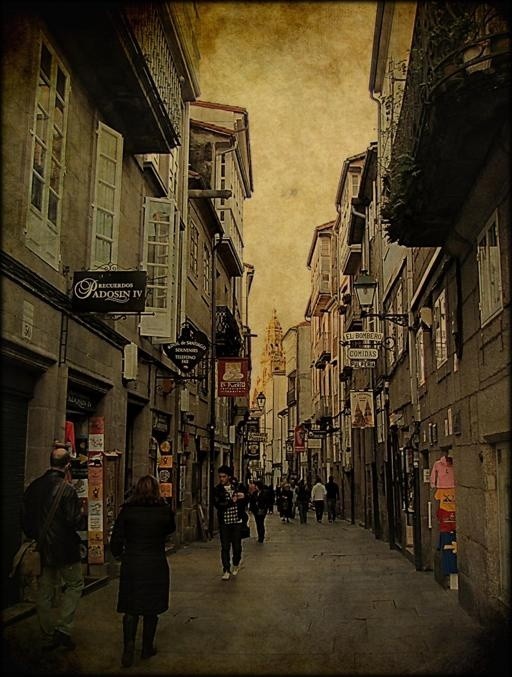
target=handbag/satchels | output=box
[9,539,41,578]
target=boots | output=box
[121,615,139,667]
[141,618,158,659]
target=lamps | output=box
[249,391,266,412]
[354,269,409,326]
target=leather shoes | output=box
[53,629,76,648]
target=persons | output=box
[211,473,343,545]
[211,462,250,582]
[108,472,178,666]
[21,445,88,648]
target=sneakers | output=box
[232,566,239,576]
[221,571,230,580]
